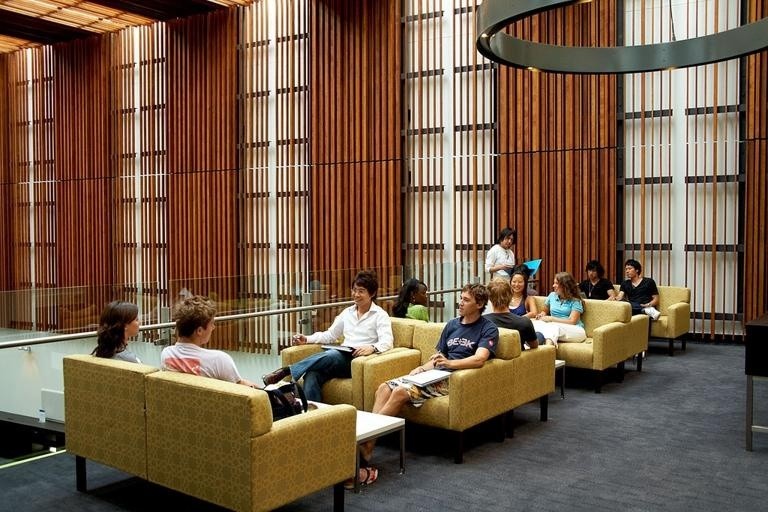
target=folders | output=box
[505,259,543,277]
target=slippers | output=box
[343,467,378,490]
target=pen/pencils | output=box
[293,335,298,344]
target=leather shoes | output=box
[262,368,286,386]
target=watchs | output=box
[648,303,652,307]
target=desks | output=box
[744,310,768,451]
[322,406,415,476]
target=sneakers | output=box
[644,307,660,320]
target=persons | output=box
[160,295,376,490]
[615,259,660,315]
[89,300,143,363]
[481,278,539,351]
[533,272,587,350]
[263,271,394,402]
[510,264,539,296]
[485,228,516,282]
[577,260,616,301]
[392,278,430,320]
[358,283,499,462]
[508,271,537,319]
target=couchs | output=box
[298,307,563,429]
[643,285,691,356]
[63,356,355,512]
[502,296,651,382]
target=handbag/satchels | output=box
[263,380,307,422]
